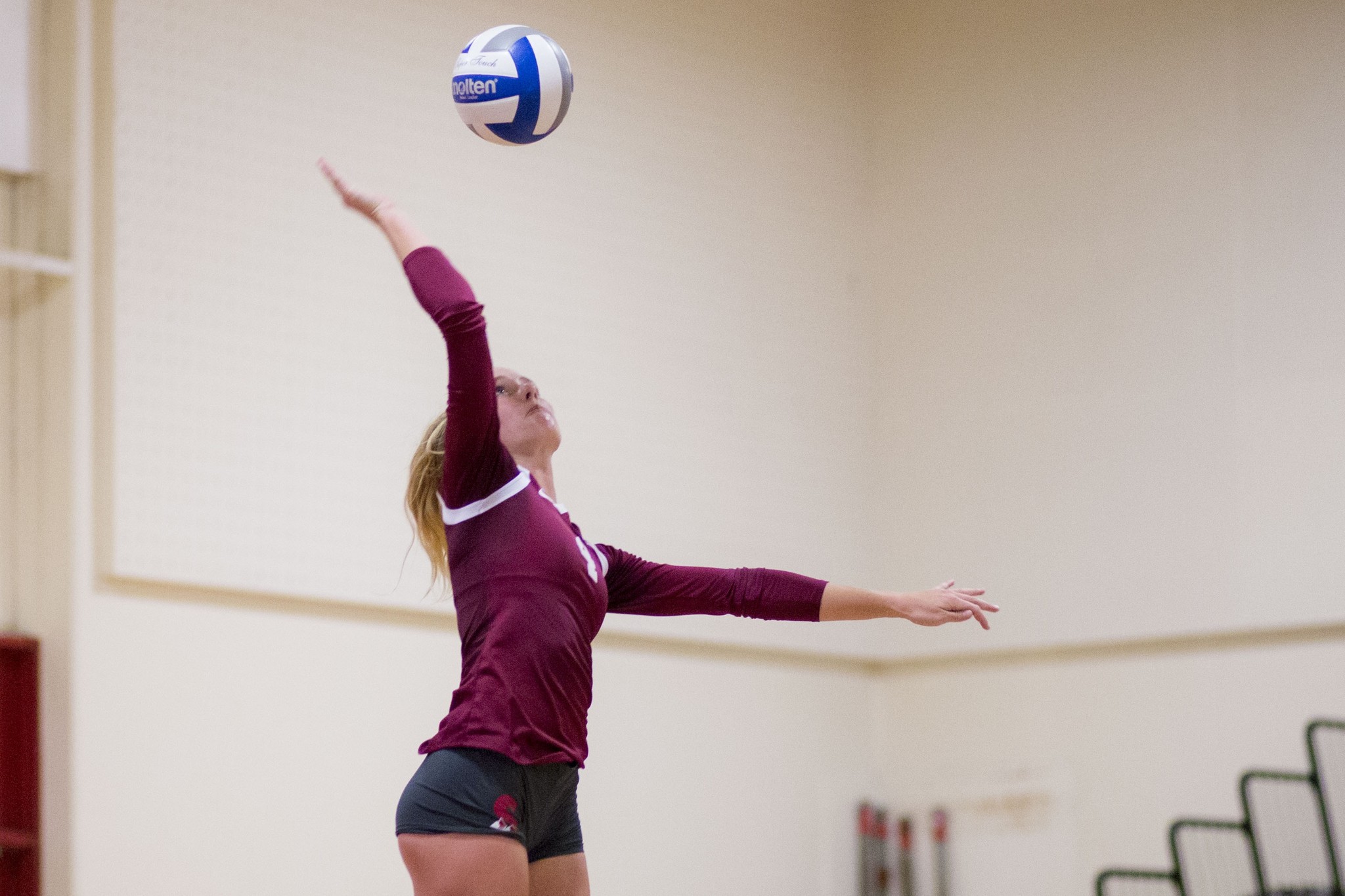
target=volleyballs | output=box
[451,24,573,146]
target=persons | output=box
[322,159,1000,896]
